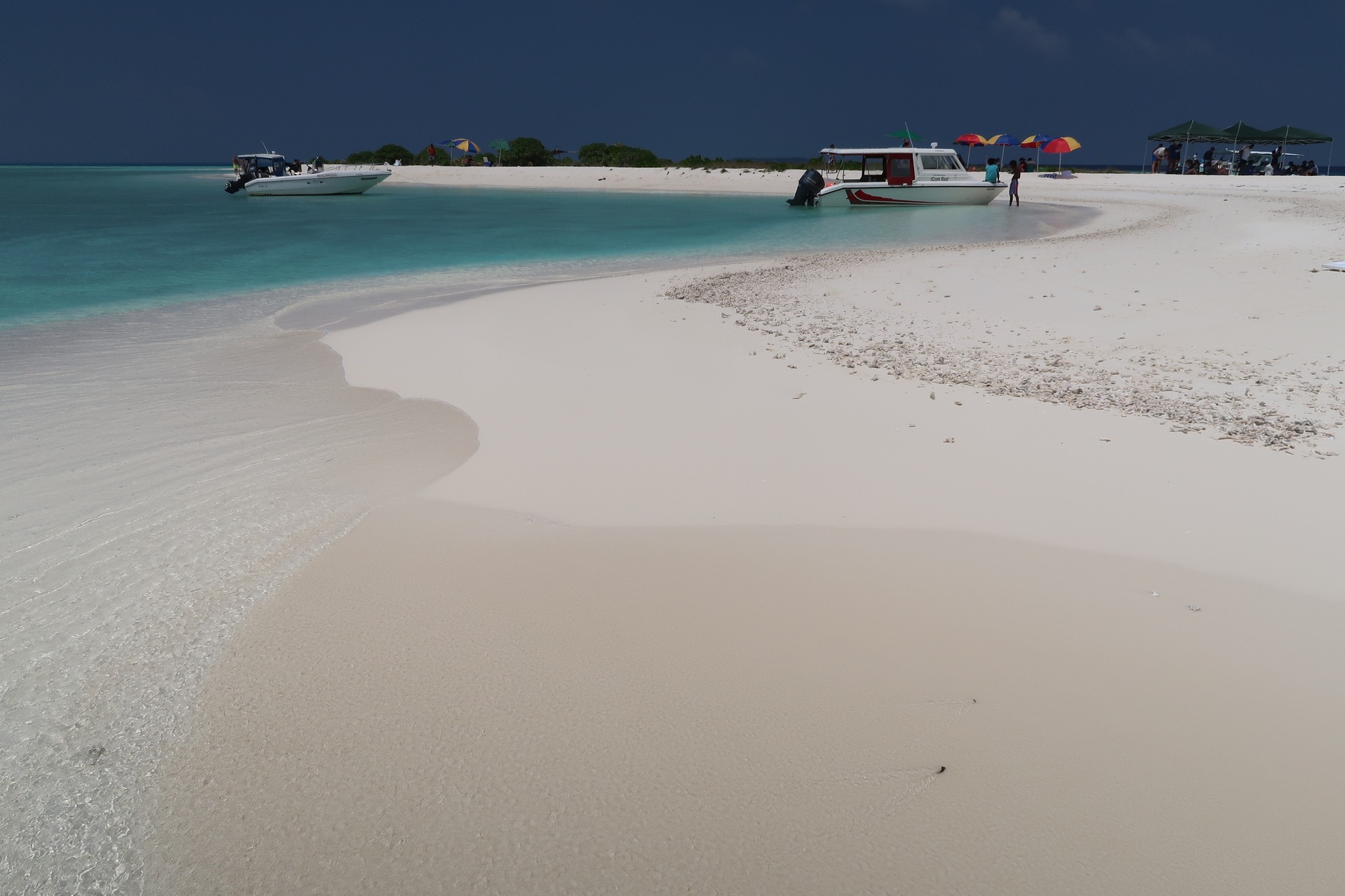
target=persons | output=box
[995,158,1004,171]
[1007,160,1021,206]
[233,156,238,175]
[826,144,838,174]
[957,154,965,168]
[427,144,438,167]
[1150,143,1318,175]
[293,159,304,176]
[985,158,1000,183]
[1017,157,1036,173]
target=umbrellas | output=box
[437,140,457,166]
[448,138,481,166]
[884,130,926,144]
[1020,134,1052,172]
[1039,137,1082,174]
[952,133,988,169]
[487,139,513,166]
[984,134,1021,172]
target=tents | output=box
[1142,120,1235,176]
[1266,126,1334,176]
[1221,121,1285,175]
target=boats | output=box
[1176,148,1304,175]
[223,139,392,196]
[784,121,1008,208]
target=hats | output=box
[1307,160,1314,163]
[1288,162,1293,166]
[1302,160,1307,164]
[1211,147,1216,150]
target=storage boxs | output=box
[483,157,491,167]
